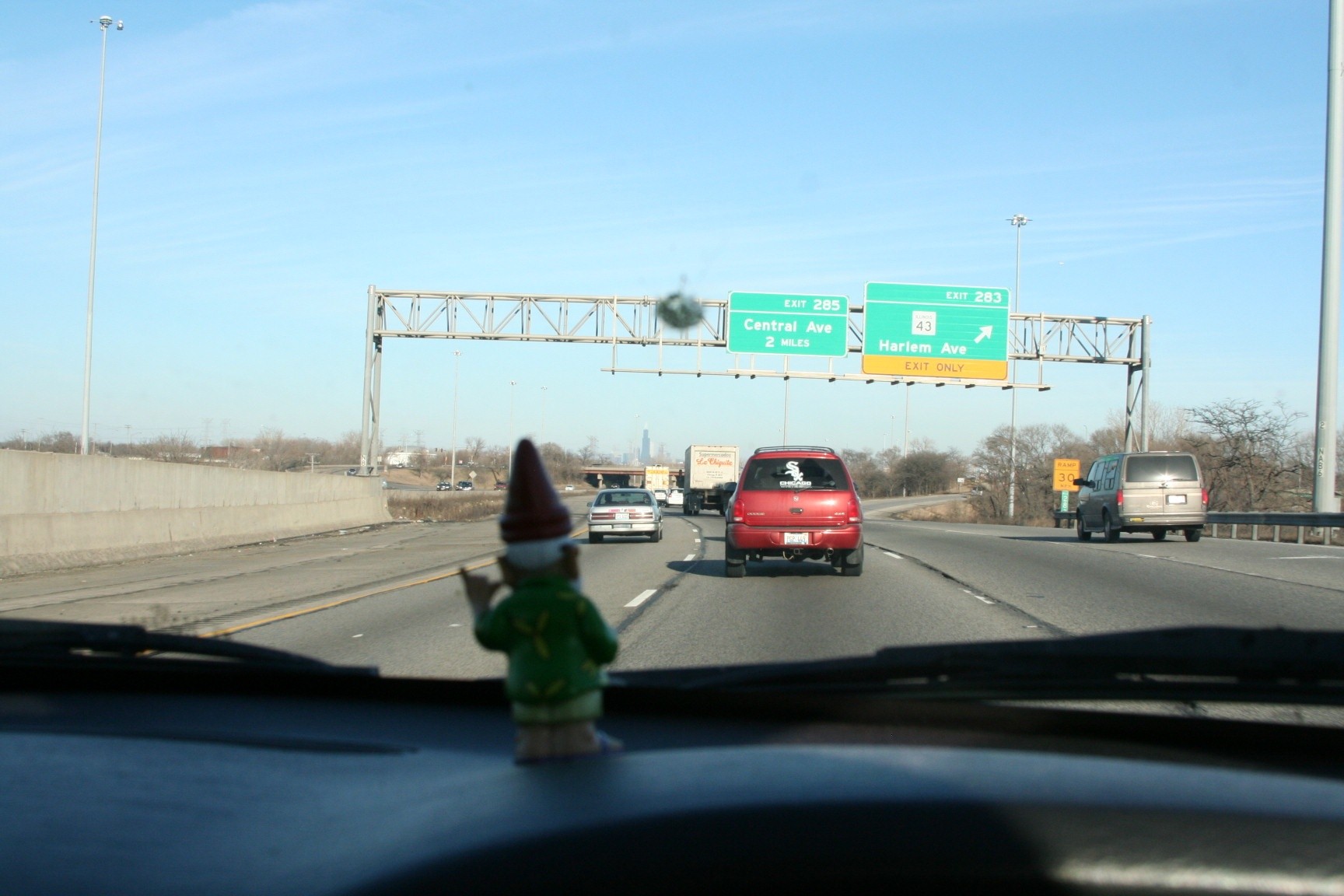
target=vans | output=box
[972,486,983,495]
[1073,450,1208,543]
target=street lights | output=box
[451,347,460,486]
[541,386,546,452]
[509,380,516,479]
[80,16,123,454]
[1006,216,1032,517]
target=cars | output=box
[397,464,403,468]
[718,481,738,515]
[587,487,664,543]
[565,485,574,490]
[349,468,355,474]
[494,481,509,490]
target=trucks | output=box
[679,444,739,515]
[644,463,669,490]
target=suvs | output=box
[666,488,685,507]
[653,489,667,505]
[724,444,864,577]
[436,481,474,491]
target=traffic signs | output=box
[862,282,1009,380]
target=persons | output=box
[461,439,623,763]
